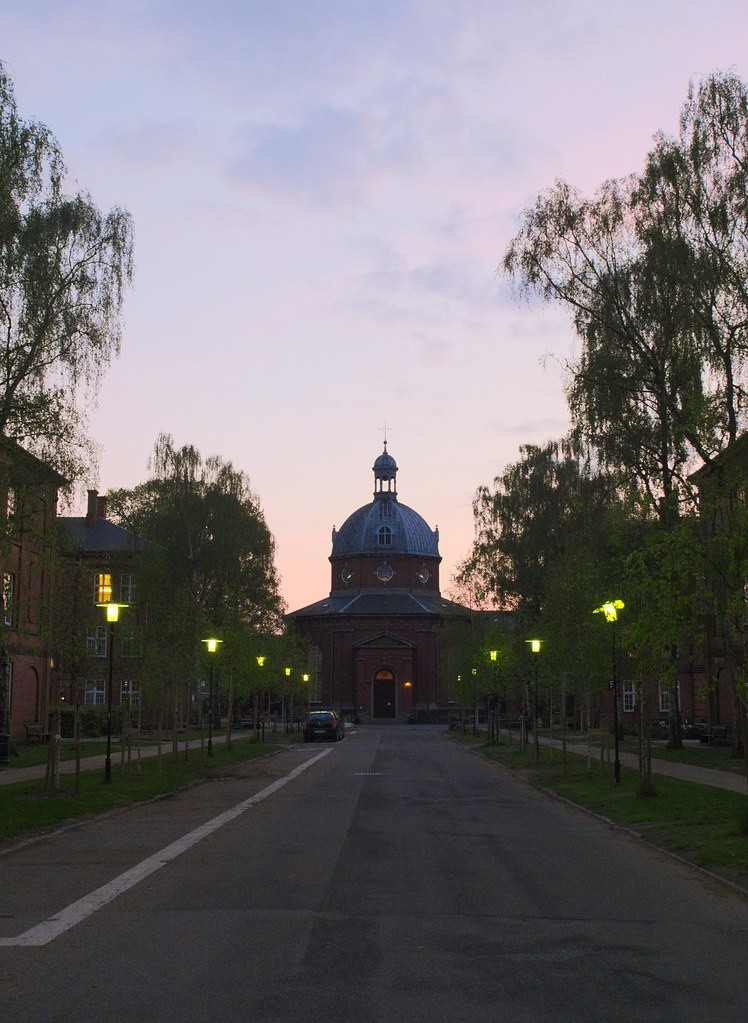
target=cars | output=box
[302,708,344,742]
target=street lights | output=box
[256,655,270,744]
[201,637,225,757]
[281,666,297,740]
[300,672,313,724]
[594,598,628,782]
[521,637,548,759]
[489,647,506,741]
[100,603,131,784]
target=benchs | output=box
[23,724,50,750]
[699,726,729,747]
